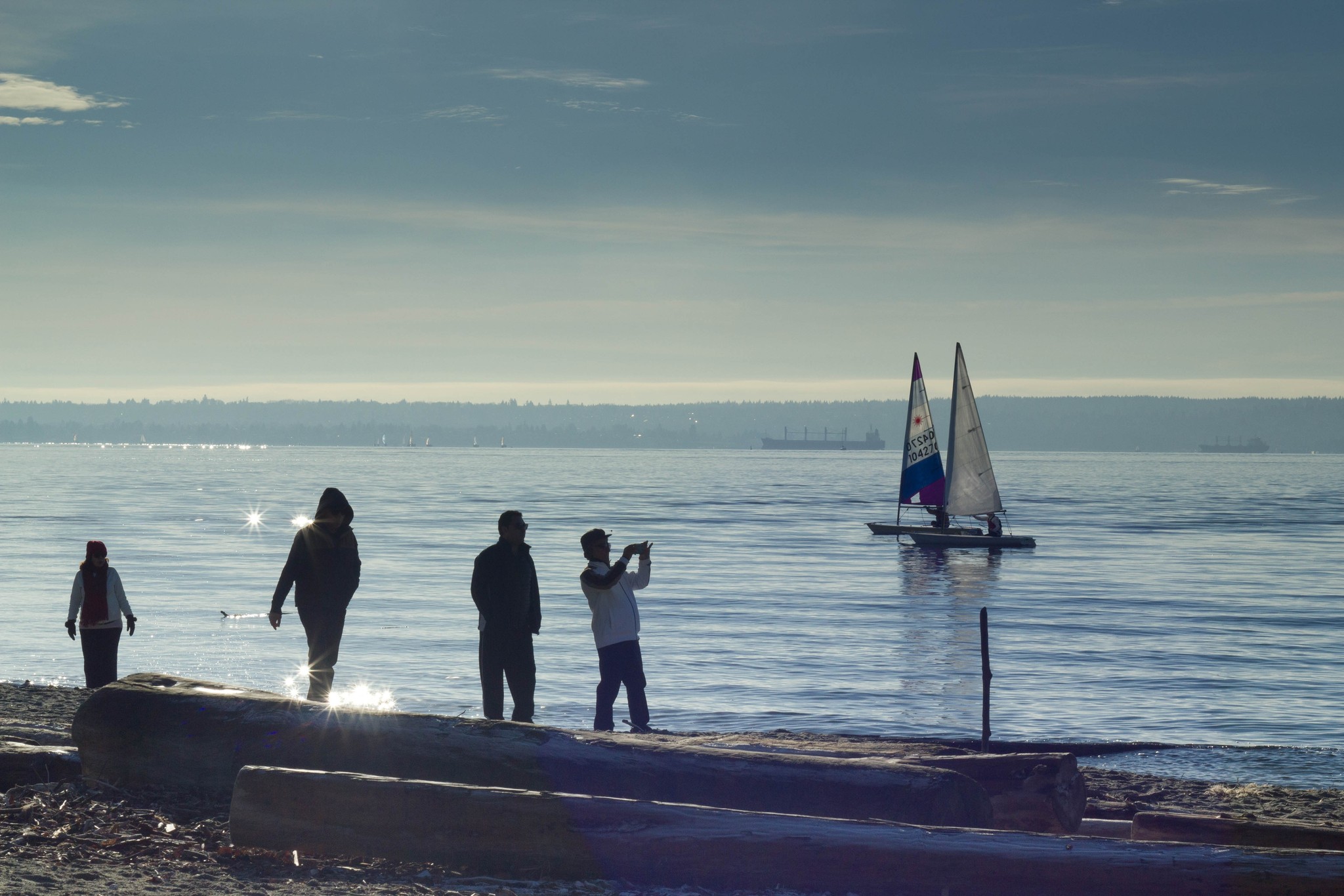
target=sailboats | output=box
[863,350,983,537]
[903,342,1038,550]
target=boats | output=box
[760,425,886,450]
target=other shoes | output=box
[631,726,652,732]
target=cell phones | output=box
[634,544,646,554]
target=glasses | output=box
[594,543,610,550]
[332,510,347,516]
[91,554,106,558]
[507,523,529,530]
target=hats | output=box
[580,529,612,548]
[86,541,107,557]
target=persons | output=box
[579,528,656,734]
[471,510,541,724]
[923,505,950,528]
[972,512,1003,537]
[65,541,137,689]
[268,487,361,702]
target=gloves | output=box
[126,615,137,636]
[65,620,76,641]
